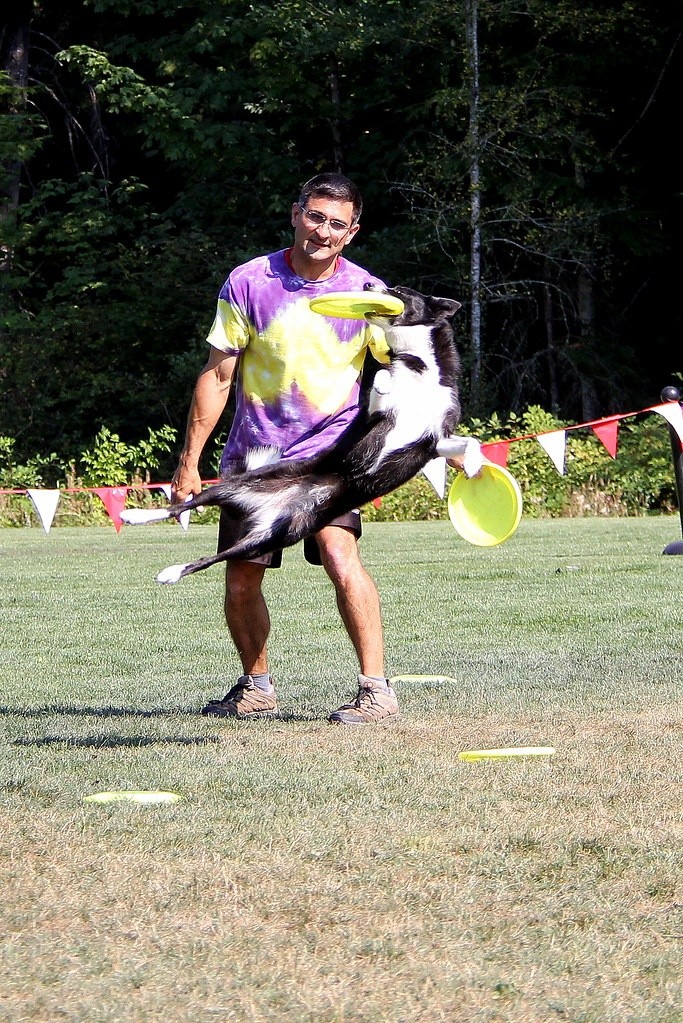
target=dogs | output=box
[118,282,482,588]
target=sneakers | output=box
[327,674,399,726]
[201,675,278,719]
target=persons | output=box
[171,173,398,723]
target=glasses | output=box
[299,207,351,231]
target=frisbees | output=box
[309,291,404,320]
[447,463,523,547]
